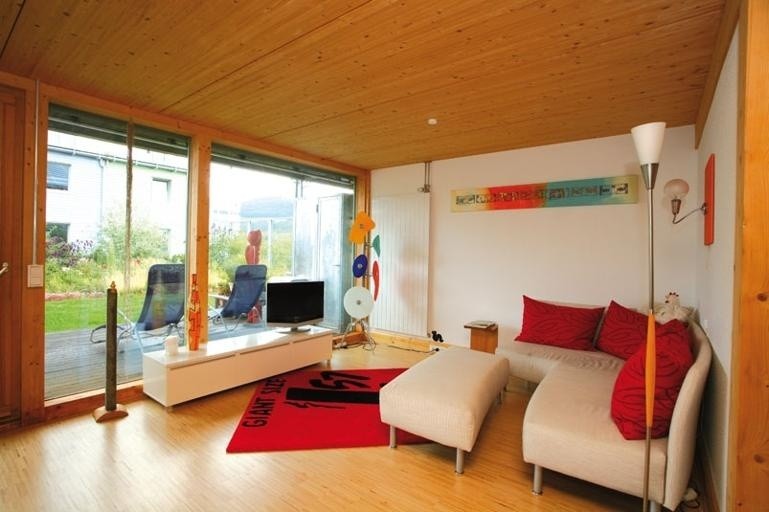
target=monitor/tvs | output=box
[266,281,324,334]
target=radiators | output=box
[364,192,431,339]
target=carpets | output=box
[227,367,435,453]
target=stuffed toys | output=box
[654,291,692,326]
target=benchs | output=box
[379,347,510,474]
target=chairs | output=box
[89,263,185,353]
[209,264,267,338]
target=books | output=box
[466,320,494,329]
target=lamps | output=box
[664,153,715,245]
[630,122,667,512]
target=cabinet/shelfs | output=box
[292,194,354,333]
[142,324,333,411]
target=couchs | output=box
[502,299,712,512]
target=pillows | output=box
[513,294,695,440]
